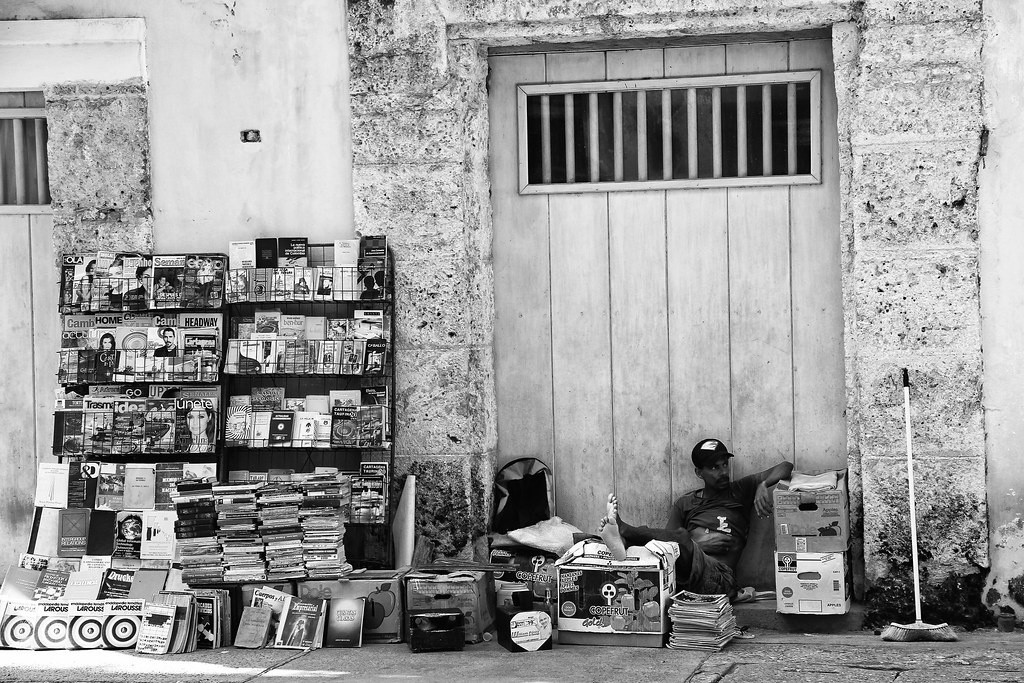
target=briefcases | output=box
[404,607,465,654]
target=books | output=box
[0,462,392,649]
[227,310,385,380]
[227,385,392,452]
[61,254,222,314]
[225,240,386,305]
[58,314,222,384]
[47,385,220,460]
[667,584,739,655]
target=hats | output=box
[691,438,735,467]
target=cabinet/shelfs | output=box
[57,243,397,585]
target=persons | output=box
[359,269,383,300]
[125,264,151,309]
[153,326,177,357]
[153,273,176,300]
[104,258,124,302]
[181,397,216,456]
[75,259,96,302]
[286,617,310,647]
[92,333,119,381]
[319,275,333,295]
[599,437,796,594]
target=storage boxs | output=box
[406,607,466,654]
[405,571,498,645]
[242,584,292,610]
[297,578,402,643]
[496,604,553,652]
[554,564,676,647]
[773,473,852,615]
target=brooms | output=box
[879,366,959,643]
